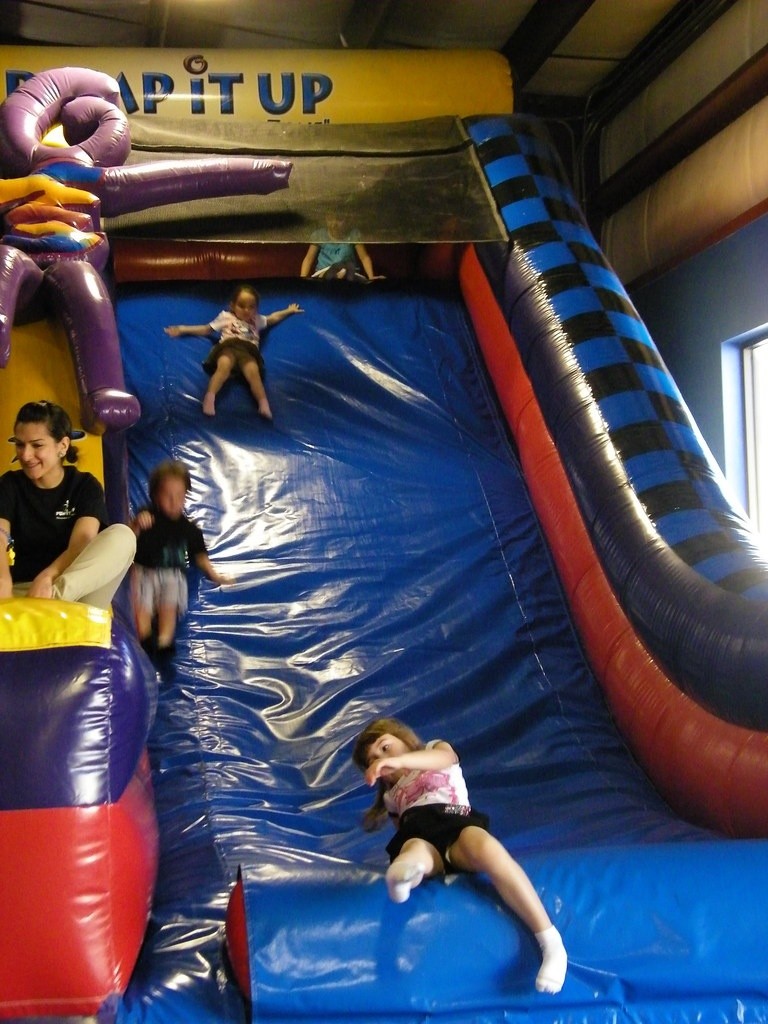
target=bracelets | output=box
[0,527,12,543]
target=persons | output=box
[164,284,304,419]
[299,204,387,284]
[0,400,136,609]
[131,460,233,678]
[352,717,568,994]
